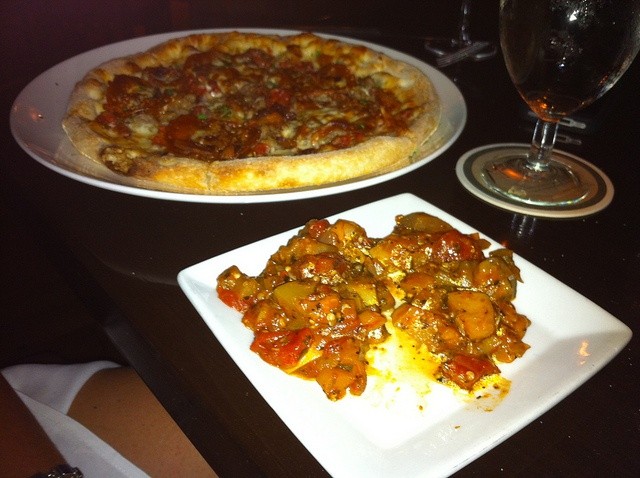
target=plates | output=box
[9,29,469,203]
[177,191,633,478]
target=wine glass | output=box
[480,0,639,208]
[424,0,497,62]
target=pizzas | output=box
[60,30,441,194]
